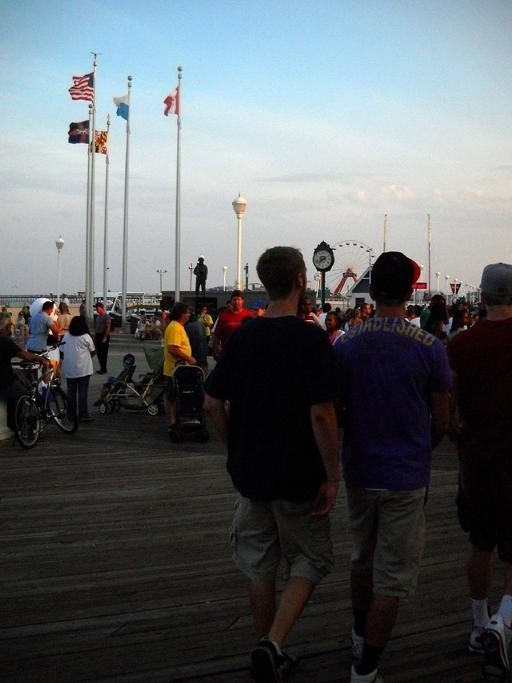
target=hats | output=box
[93,301,105,308]
[479,261,512,294]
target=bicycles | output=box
[15,340,78,448]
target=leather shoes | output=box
[97,370,100,372]
[100,367,106,374]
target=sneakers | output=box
[252,635,290,682]
[479,612,509,683]
[351,637,364,661]
[467,623,486,654]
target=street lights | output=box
[454,278,475,303]
[435,270,440,292]
[231,191,248,291]
[222,264,228,290]
[157,267,167,291]
[419,264,423,301]
[445,274,448,304]
[188,262,195,291]
[54,234,66,306]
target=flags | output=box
[68,120,89,143]
[163,86,180,116]
[90,130,108,154]
[114,98,129,120]
[69,73,94,102]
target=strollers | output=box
[93,337,165,416]
[168,357,209,442]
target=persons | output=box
[446,262,512,681]
[94,294,488,446]
[203,246,342,683]
[331,252,452,682]
[1,298,110,440]
[193,258,208,295]
